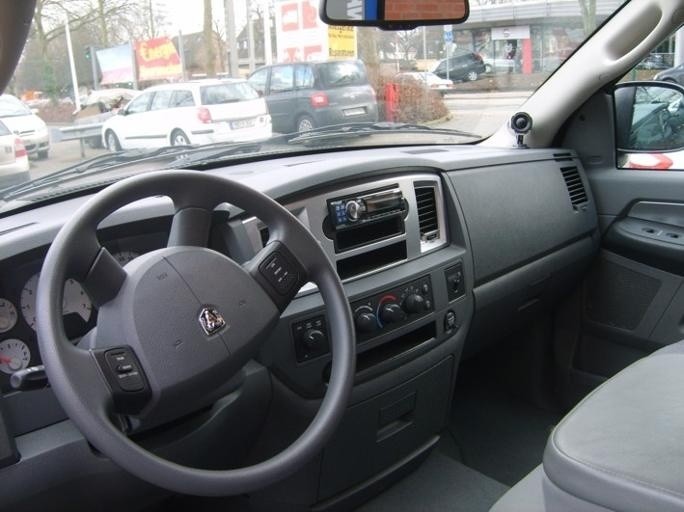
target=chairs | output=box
[544,337,683,512]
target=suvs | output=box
[433,53,486,81]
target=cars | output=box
[0,94,51,187]
[390,69,453,98]
[652,62,684,87]
[101,76,273,159]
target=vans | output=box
[247,58,378,146]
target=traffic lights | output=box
[84,46,91,59]
